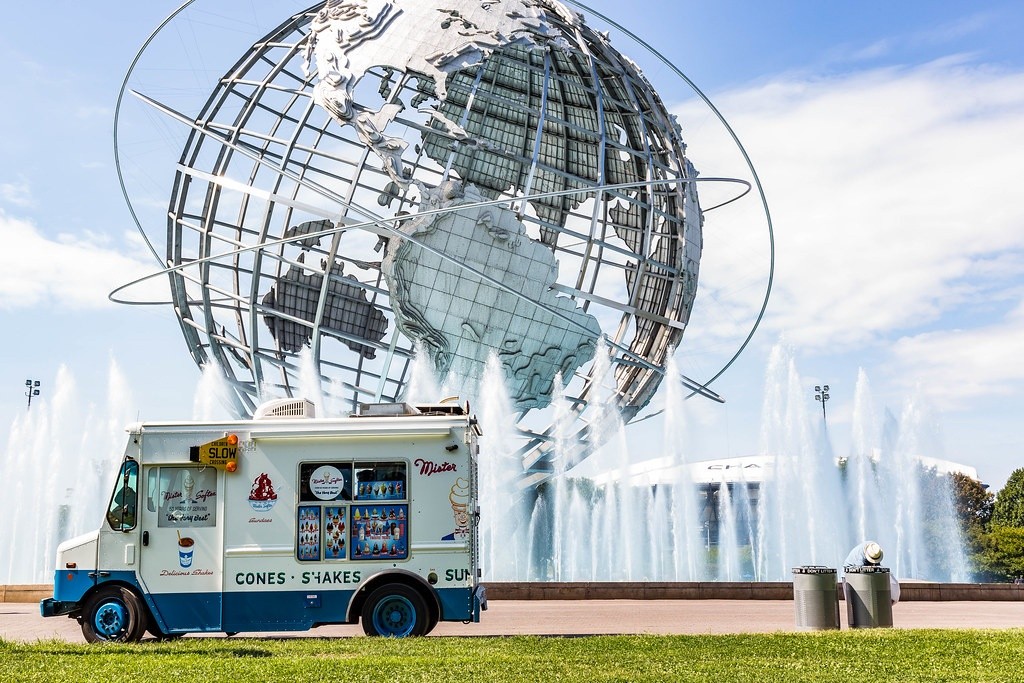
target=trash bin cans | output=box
[844,564,894,628]
[792,563,840,633]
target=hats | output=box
[864,543,883,563]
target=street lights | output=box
[815,383,830,423]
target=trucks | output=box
[38,394,489,647]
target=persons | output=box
[842,541,883,593]
